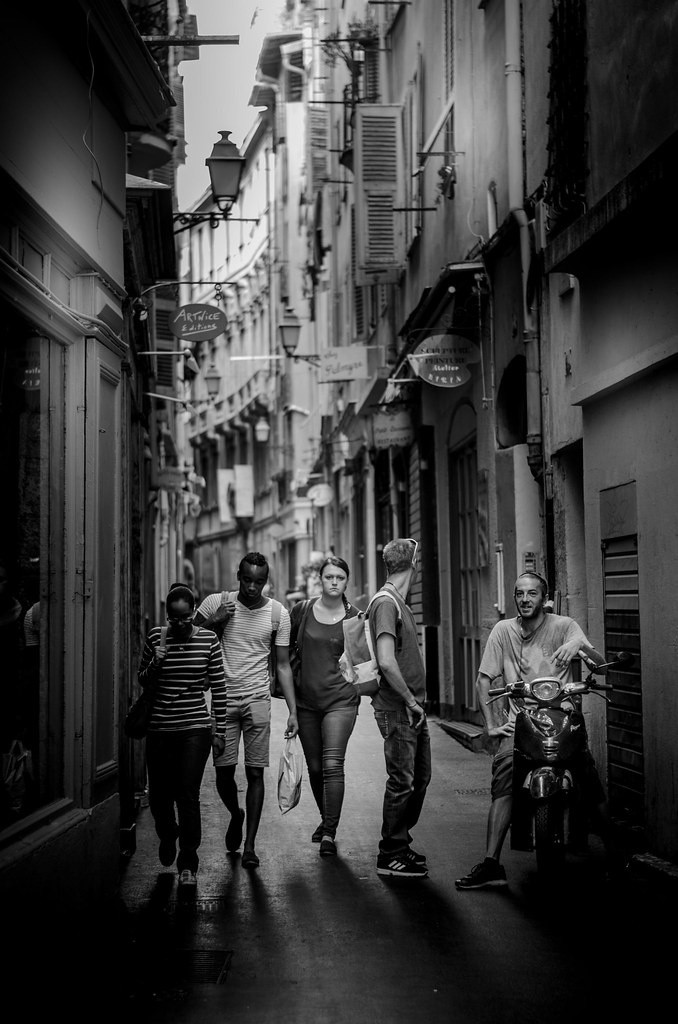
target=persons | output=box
[192,552,298,867]
[287,556,366,857]
[362,537,432,876]
[137,583,226,887]
[0,565,41,648]
[454,572,608,889]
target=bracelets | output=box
[214,734,226,739]
[409,703,417,708]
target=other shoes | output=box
[242,851,259,866]
[226,808,245,851]
[321,840,336,855]
[311,825,323,842]
[159,822,178,867]
[177,847,199,875]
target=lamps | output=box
[172,130,247,235]
[277,306,322,368]
[255,415,294,458]
[174,362,223,415]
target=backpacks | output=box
[339,590,401,695]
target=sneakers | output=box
[377,855,428,877]
[455,857,507,890]
[405,849,426,865]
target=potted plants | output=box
[347,16,381,38]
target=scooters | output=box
[482,648,636,898]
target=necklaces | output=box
[386,582,405,601]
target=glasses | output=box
[406,539,418,563]
[167,615,192,623]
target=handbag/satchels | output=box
[271,600,312,699]
[277,732,304,814]
[124,688,154,739]
